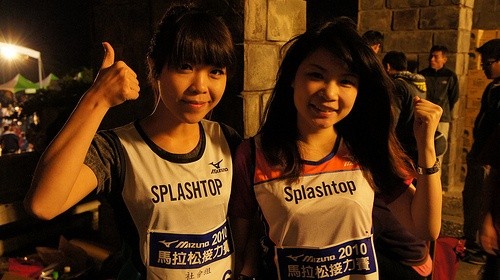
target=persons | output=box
[417,45,459,180]
[455,38,500,265]
[1,89,39,151]
[382,50,426,188]
[362,30,384,54]
[477,164,500,280]
[228,15,443,280]
[22,2,260,280]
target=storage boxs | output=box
[459,29,500,180]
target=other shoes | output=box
[468,253,488,265]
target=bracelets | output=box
[418,158,441,176]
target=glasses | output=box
[480,60,498,67]
